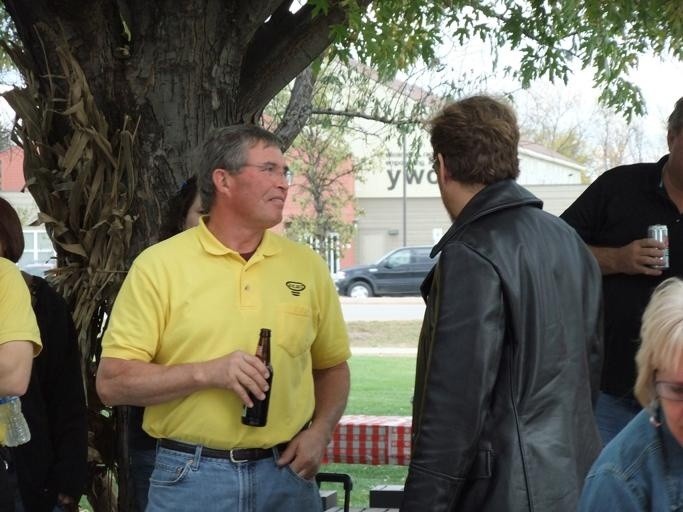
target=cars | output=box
[334,245,441,298]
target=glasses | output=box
[241,165,294,179]
[655,382,683,400]
[432,158,440,174]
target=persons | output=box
[399,95,603,512]
[577,277,683,512]
[0,256,43,512]
[558,97,683,449]
[95,124,351,512]
[0,197,89,512]
[132,175,203,512]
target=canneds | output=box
[647,224,670,270]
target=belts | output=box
[159,438,290,463]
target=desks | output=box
[327,414,415,466]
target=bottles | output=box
[0,392,32,448]
[239,328,274,426]
